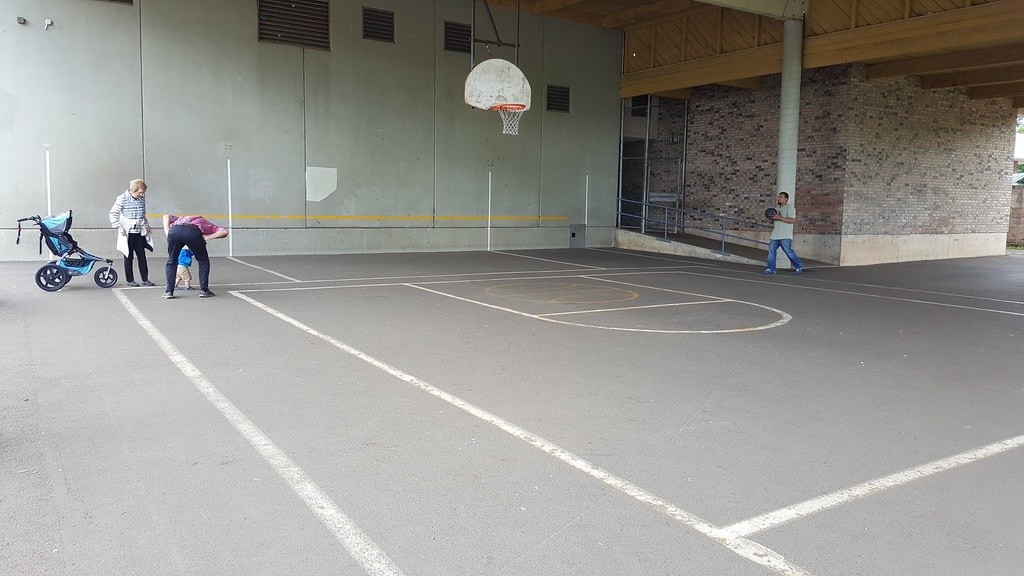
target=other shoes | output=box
[185,286,194,290]
[174,286,180,289]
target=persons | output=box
[108,179,156,286]
[162,214,228,299]
[762,192,805,274]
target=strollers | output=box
[17,207,117,292]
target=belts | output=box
[174,223,194,225]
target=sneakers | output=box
[763,269,776,274]
[199,289,215,296]
[162,292,173,299]
[142,280,154,286]
[128,281,139,287]
[795,266,804,275]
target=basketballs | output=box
[765,208,777,219]
